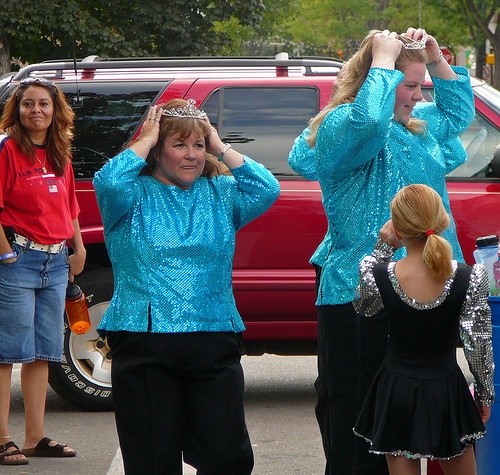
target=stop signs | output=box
[440,47,452,65]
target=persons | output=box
[352,184,495,475]
[0,77,86,465]
[92,98,280,475]
[288,27,476,475]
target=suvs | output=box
[1,51,499,411]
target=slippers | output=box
[21,437,76,457]
[0,441,29,465]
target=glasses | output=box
[20,77,54,86]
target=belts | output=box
[9,233,66,254]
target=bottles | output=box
[473,234,500,297]
[66,279,91,335]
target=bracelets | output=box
[218,143,231,161]
[0,252,17,260]
[426,50,443,67]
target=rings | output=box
[148,117,155,120]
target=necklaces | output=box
[35,153,47,174]
